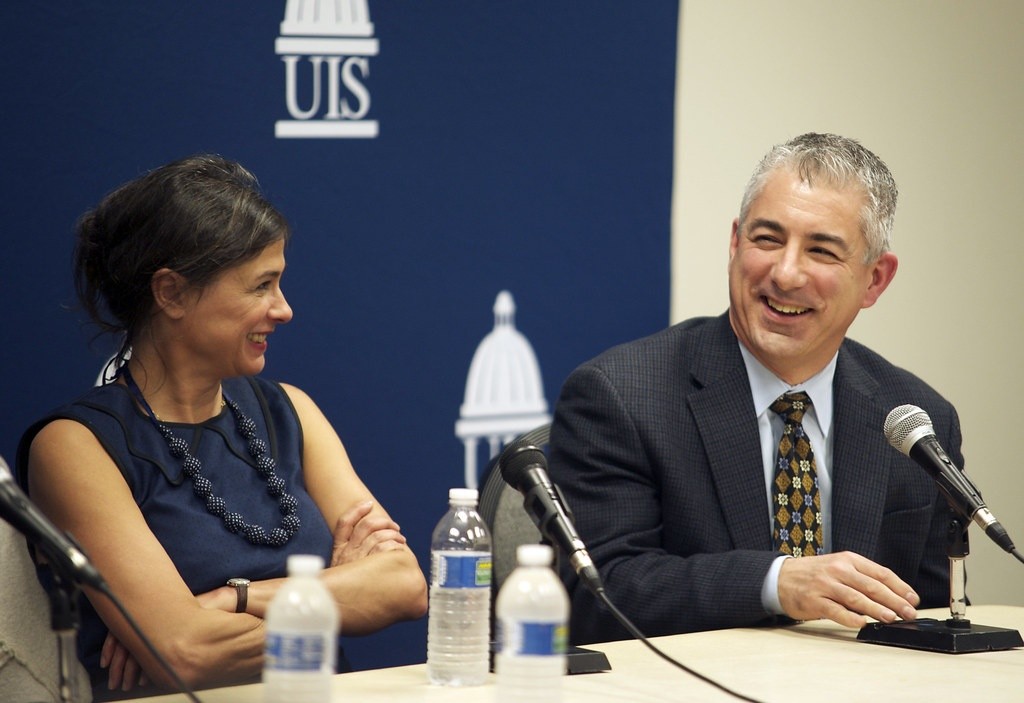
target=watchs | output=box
[227,577,251,613]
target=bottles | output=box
[497,544,568,703]
[263,555,338,703]
[427,488,492,688]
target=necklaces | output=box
[121,360,301,545]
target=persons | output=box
[546,132,971,644]
[10,154,429,703]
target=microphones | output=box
[497,438,606,598]
[0,454,103,591]
[883,404,1016,554]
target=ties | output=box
[768,391,826,557]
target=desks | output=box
[118,604,1024,703]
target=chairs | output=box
[0,417,554,703]
